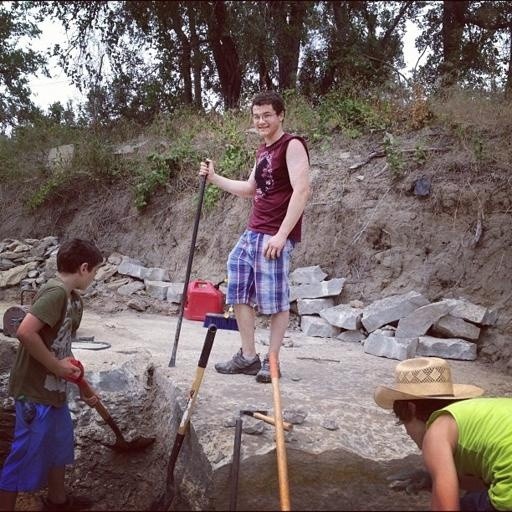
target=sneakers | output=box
[215,349,261,375]
[256,359,281,382]
[45,495,93,512]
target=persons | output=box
[372,357,512,512]
[0,237,104,512]
[199,93,311,383]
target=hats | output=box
[375,357,484,410]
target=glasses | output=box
[251,113,276,121]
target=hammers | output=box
[240,410,293,432]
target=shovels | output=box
[62,359,157,450]
[155,323,217,512]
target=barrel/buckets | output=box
[182,279,226,321]
